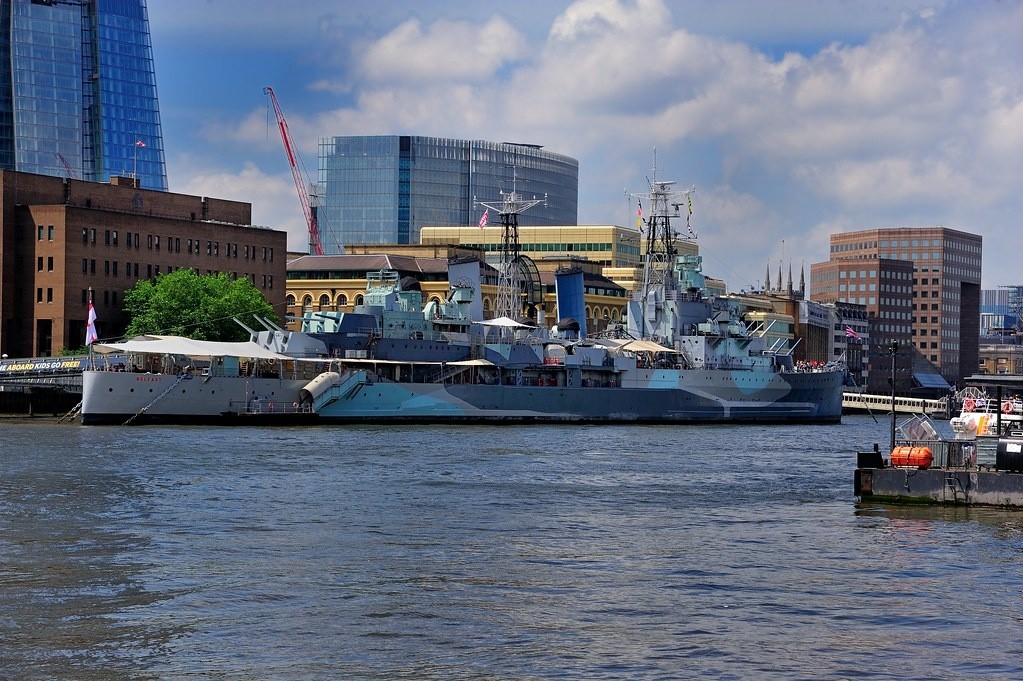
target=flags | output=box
[136,136,146,148]
[479,209,489,227]
[846,325,861,341]
[949,385,956,392]
[637,199,646,232]
[688,199,692,213]
[85,302,98,346]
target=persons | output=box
[269,401,274,412]
[797,360,825,373]
[292,401,300,410]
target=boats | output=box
[891,445,935,470]
[78,146,848,429]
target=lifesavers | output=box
[965,400,975,411]
[269,402,275,409]
[293,402,299,408]
[433,313,439,320]
[1003,402,1014,413]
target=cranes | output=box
[263,86,341,255]
